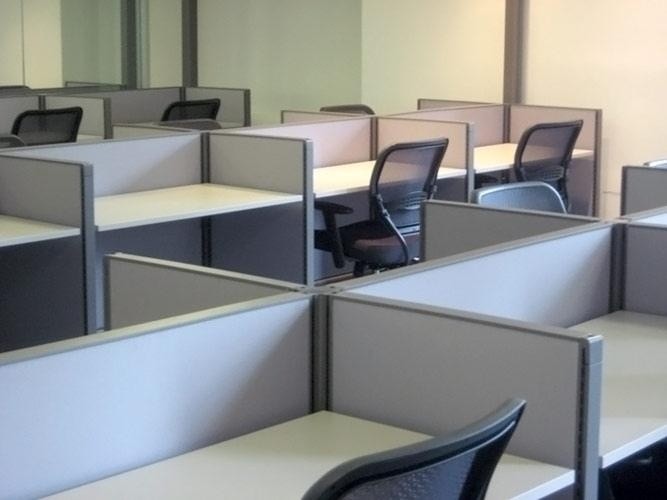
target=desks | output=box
[0,76,666,500]
